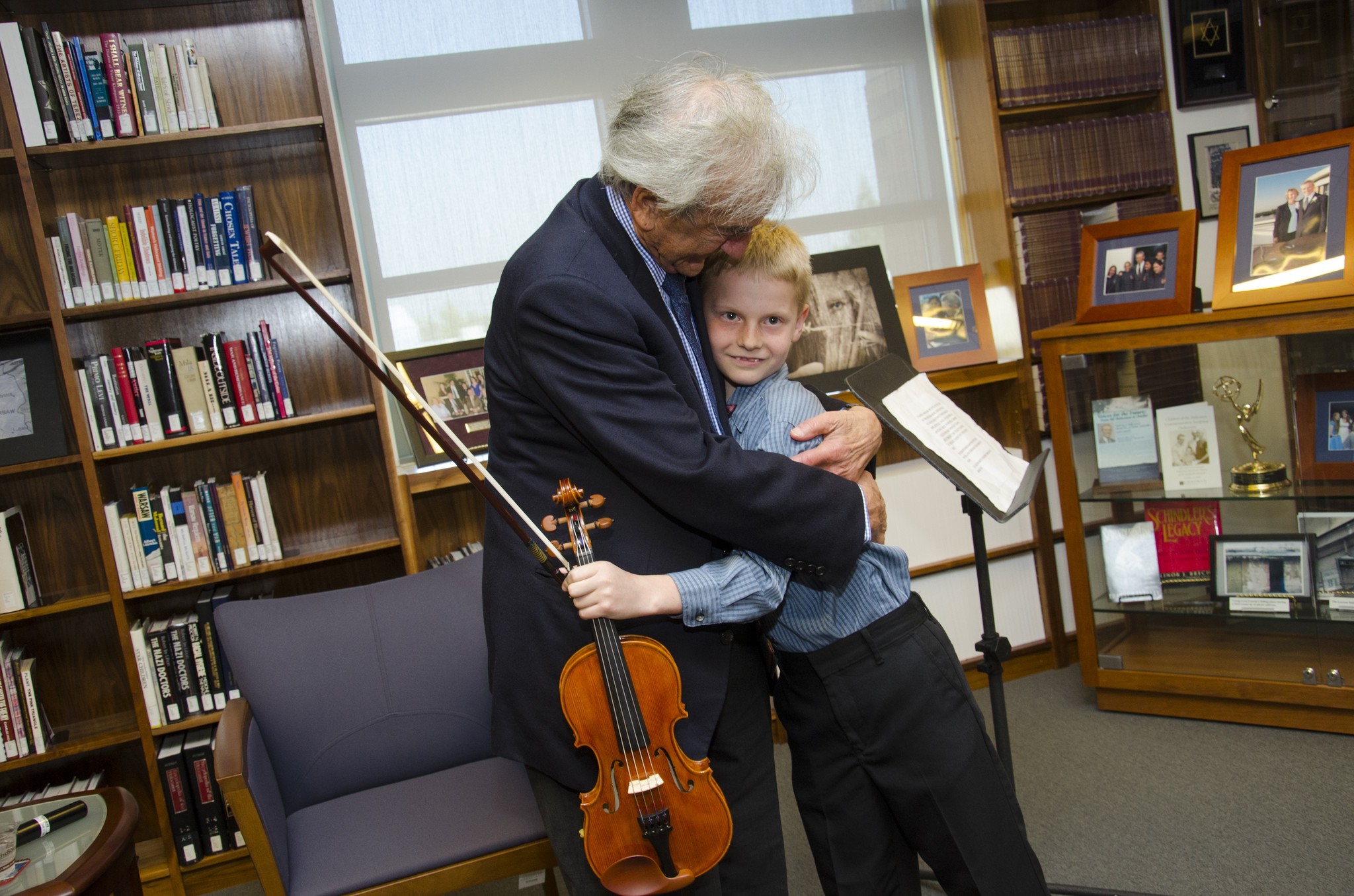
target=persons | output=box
[1169,430,1213,467]
[1329,410,1353,450]
[435,373,492,418]
[788,269,890,378]
[479,55,889,894]
[1106,249,1165,293]
[1295,180,1328,238]
[921,292,966,340]
[558,219,1051,896]
[1099,423,1116,442]
[1272,188,1300,244]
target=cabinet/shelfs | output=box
[934,0,1197,331]
[0,1,421,896]
[1029,295,1354,741]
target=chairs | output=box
[212,550,551,896]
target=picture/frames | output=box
[1208,532,1319,603]
[1210,128,1354,311]
[1065,202,1201,328]
[1167,0,1253,112]
[1187,125,1252,222]
[1290,362,1354,499]
[377,338,511,472]
[1265,0,1354,101]
[1273,113,1338,142]
[890,264,1000,373]
[781,246,911,392]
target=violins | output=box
[541,478,734,896]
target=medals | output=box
[1212,375,1293,493]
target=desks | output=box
[392,355,1073,749]
[0,783,140,896]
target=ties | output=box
[662,272,726,438]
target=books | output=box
[71,319,295,452]
[0,17,219,149]
[156,723,250,868]
[128,594,275,731]
[0,639,53,764]
[0,468,289,613]
[47,182,269,310]
